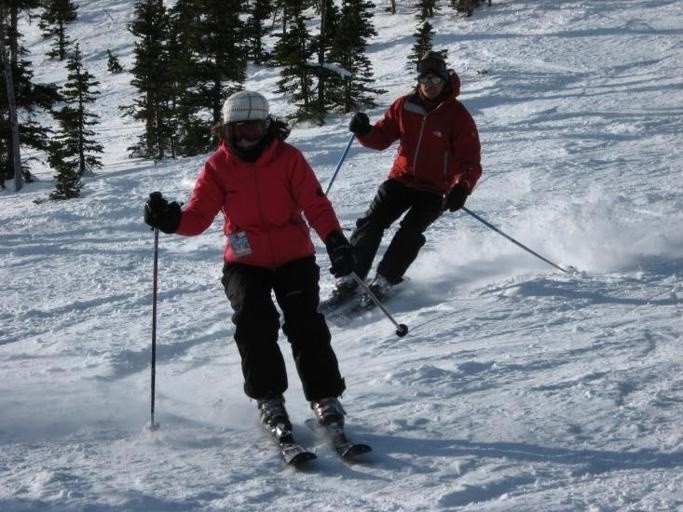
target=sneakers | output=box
[314,401,343,424]
[261,402,291,438]
[358,276,389,309]
[335,276,358,292]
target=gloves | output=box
[326,232,354,278]
[442,182,467,212]
[145,192,180,234]
[350,112,368,133]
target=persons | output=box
[144,91,359,438]
[336,51,483,308]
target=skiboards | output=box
[255,398,373,465]
[317,274,406,320]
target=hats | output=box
[419,52,446,78]
[224,91,270,122]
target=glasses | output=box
[222,123,265,141]
[418,77,442,83]
